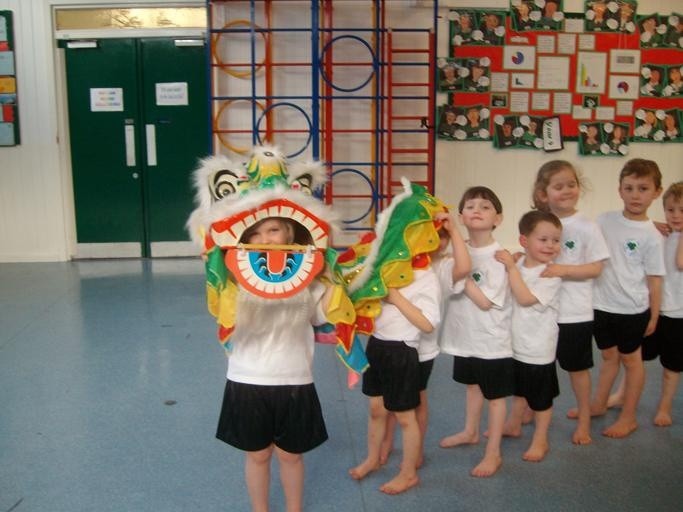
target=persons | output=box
[348,259,440,494]
[377,216,470,476]
[441,0,682,153]
[212,218,335,512]
[511,162,608,446]
[565,158,662,441]
[480,215,563,462]
[607,182,681,426]
[437,184,509,479]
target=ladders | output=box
[388,28,435,208]
